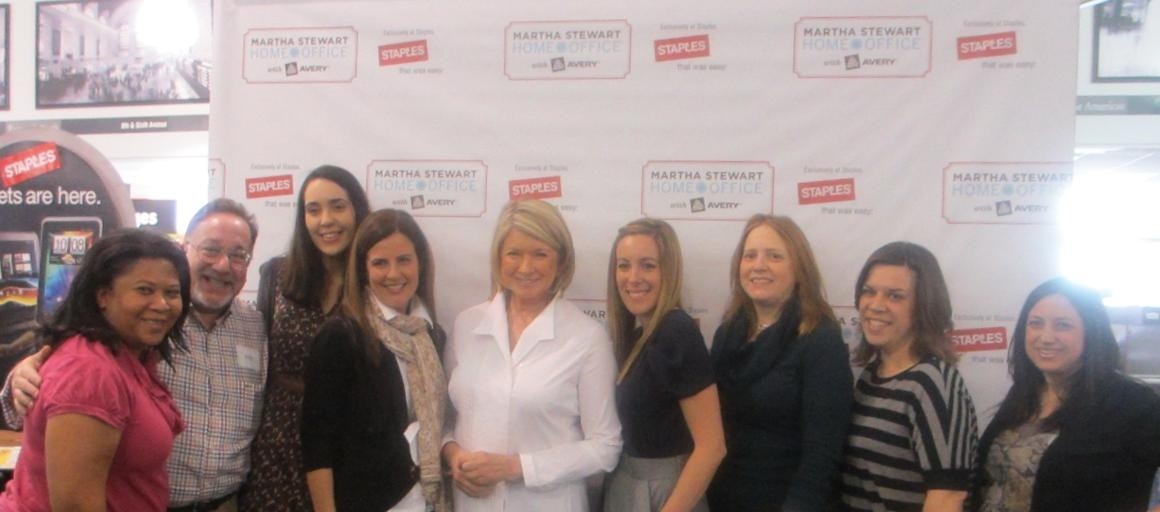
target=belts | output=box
[166,492,235,512]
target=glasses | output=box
[197,240,251,264]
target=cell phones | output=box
[35,215,103,322]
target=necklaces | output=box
[755,317,771,339]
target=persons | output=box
[979,275,1160,512]
[440,196,625,511]
[709,212,854,511]
[0,197,270,511]
[238,165,371,511]
[589,217,728,512]
[839,240,980,511]
[299,207,448,511]
[0,227,191,510]
